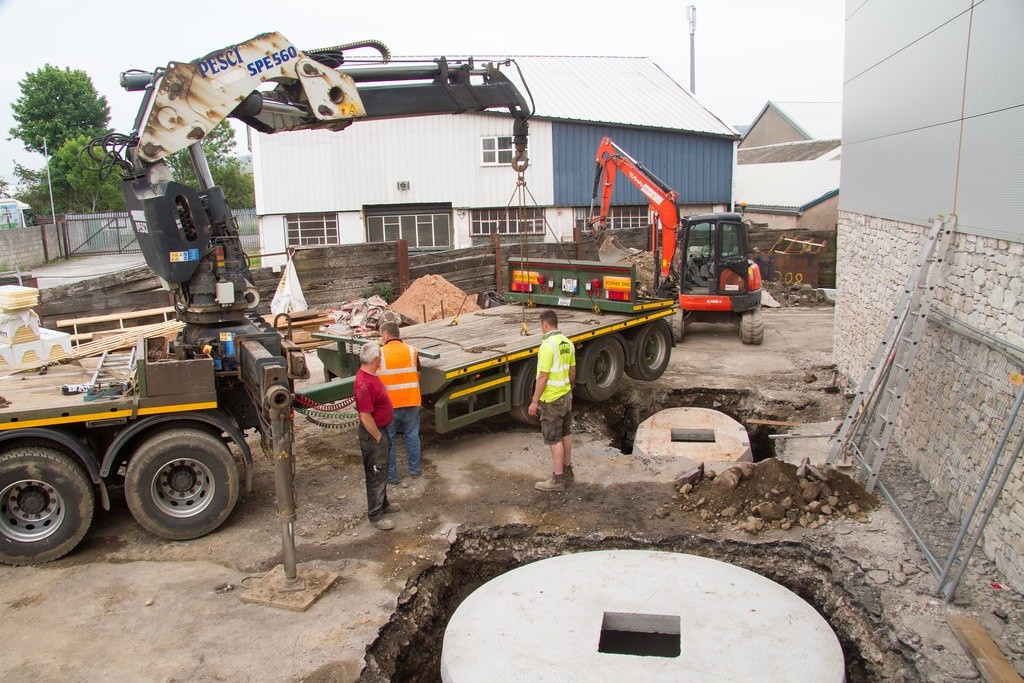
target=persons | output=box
[527,310,576,492]
[355,343,400,530]
[378,322,423,486]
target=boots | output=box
[563,463,574,482]
[535,472,565,491]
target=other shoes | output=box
[412,473,421,479]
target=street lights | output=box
[686,4,698,96]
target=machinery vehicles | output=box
[583,135,762,348]
[0,29,535,614]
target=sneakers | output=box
[370,517,395,529]
[382,503,401,513]
